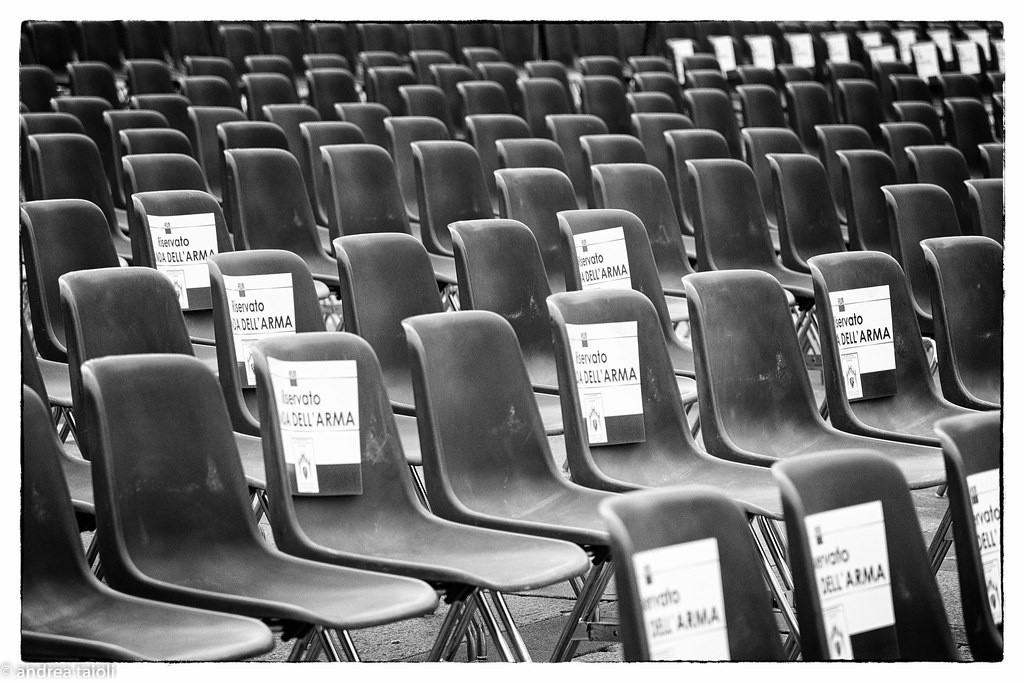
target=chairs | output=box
[19,20,1004,664]
